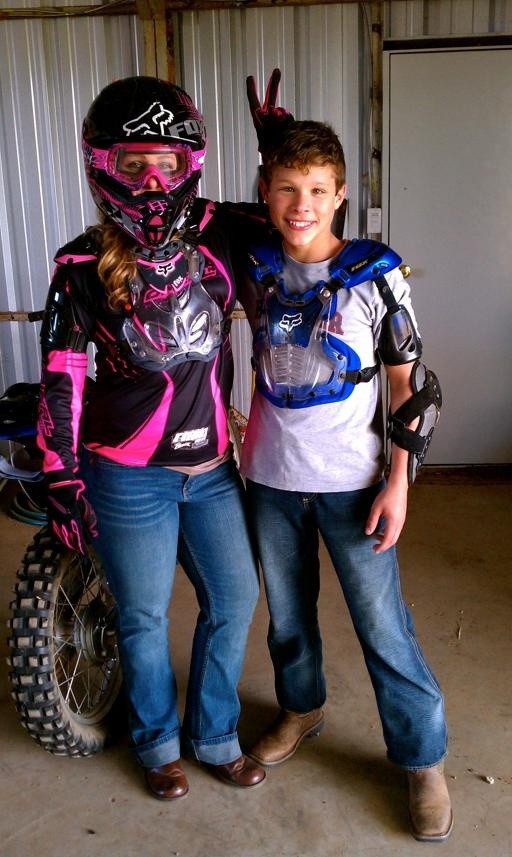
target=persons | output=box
[32,66,295,807]
[238,117,458,843]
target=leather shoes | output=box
[206,754,267,790]
[245,706,325,764]
[145,759,191,803]
[406,759,455,845]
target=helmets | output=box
[81,74,206,262]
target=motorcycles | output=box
[0,304,257,761]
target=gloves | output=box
[245,67,294,166]
[46,478,99,557]
